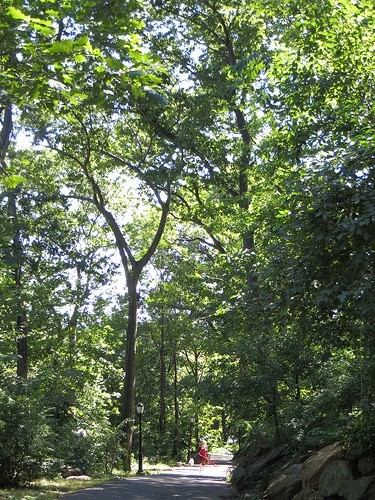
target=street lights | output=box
[136,402,146,477]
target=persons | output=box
[198,441,208,472]
